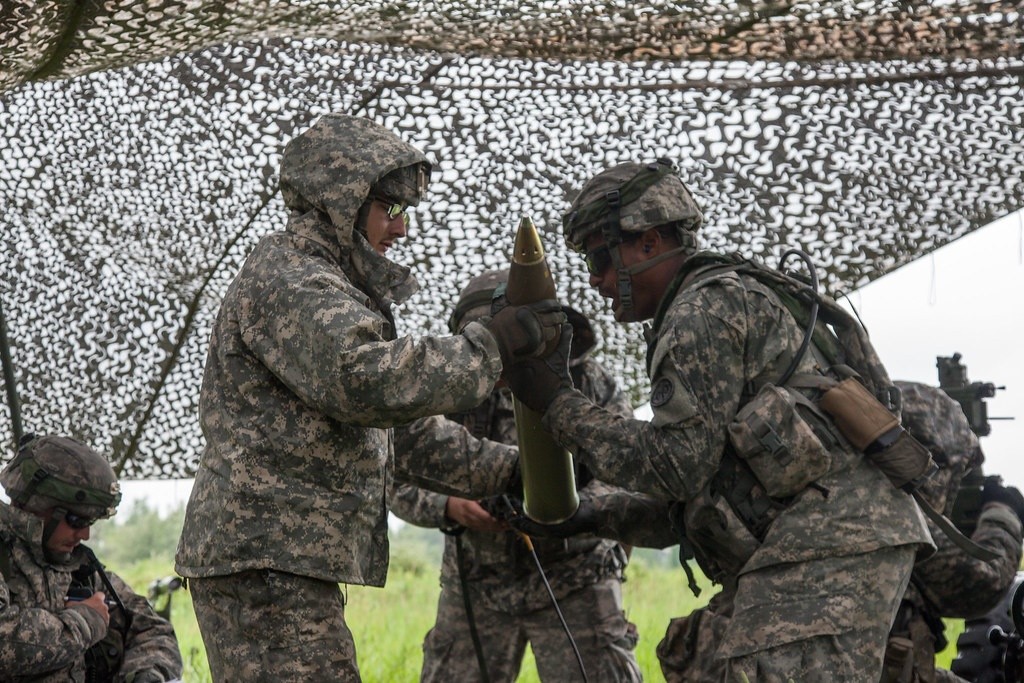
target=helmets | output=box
[449,266,511,336]
[562,157,703,254]
[367,159,430,207]
[0,433,122,518]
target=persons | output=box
[176,113,568,683]
[385,270,646,682]
[0,436,183,682]
[883,380,1023,682]
[492,161,938,683]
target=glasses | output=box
[581,235,638,277]
[372,196,410,226]
[54,506,96,529]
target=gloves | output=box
[493,321,573,415]
[493,489,595,538]
[506,453,593,505]
[482,294,567,374]
